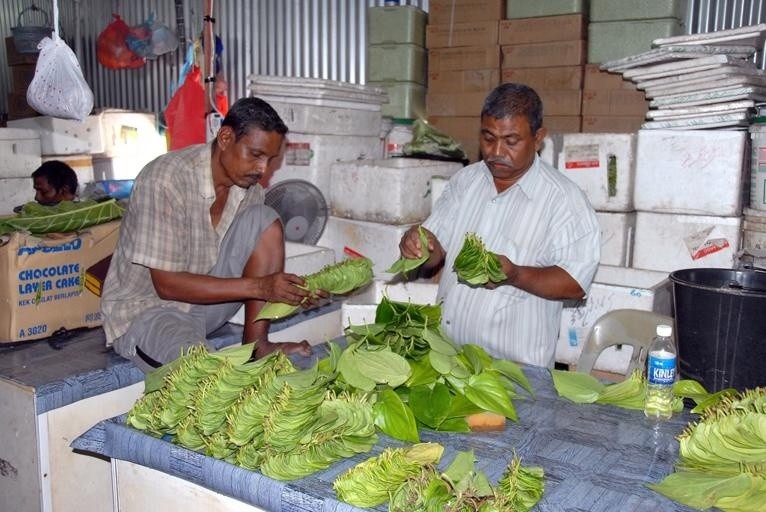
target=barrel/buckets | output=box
[669,267,765,408]
[749,124,766,211]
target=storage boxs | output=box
[1,218,123,345]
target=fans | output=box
[264,178,329,246]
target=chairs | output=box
[574,308,675,378]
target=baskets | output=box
[11,7,52,54]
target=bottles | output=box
[380,115,416,159]
[643,323,679,419]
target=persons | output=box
[399,82,602,369]
[97,96,329,377]
[12,160,82,213]
